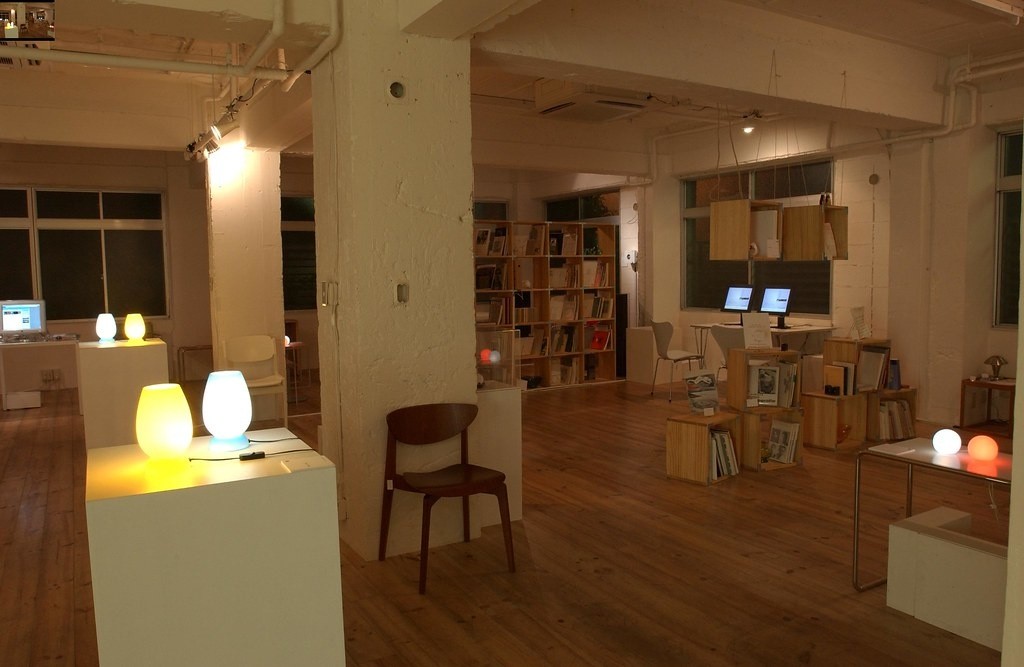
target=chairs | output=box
[223,335,288,428]
[20,24,28,34]
[710,324,745,385]
[649,318,704,403]
[378,403,516,596]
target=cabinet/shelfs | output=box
[473,219,617,393]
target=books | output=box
[475,225,614,386]
[768,420,800,464]
[824,346,902,396]
[748,361,797,409]
[709,428,740,480]
[683,369,719,416]
[880,400,915,439]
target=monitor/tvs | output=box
[0,301,47,342]
[720,285,755,327]
[757,287,792,329]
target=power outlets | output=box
[54,369,60,381]
[43,370,53,381]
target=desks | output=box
[0,20,9,27]
[4,25,18,38]
[177,341,311,387]
[887,506,1008,652]
[78,338,169,453]
[0,333,84,416]
[691,322,836,369]
[86,427,346,667]
[46,26,55,38]
[960,375,1016,438]
[852,438,1012,592]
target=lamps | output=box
[933,429,962,455]
[984,356,1008,380]
[209,139,219,149]
[135,383,194,475]
[95,313,117,345]
[202,371,254,453]
[211,120,240,140]
[742,118,754,134]
[124,313,146,344]
[967,435,999,463]
[206,145,213,154]
[208,142,216,152]
